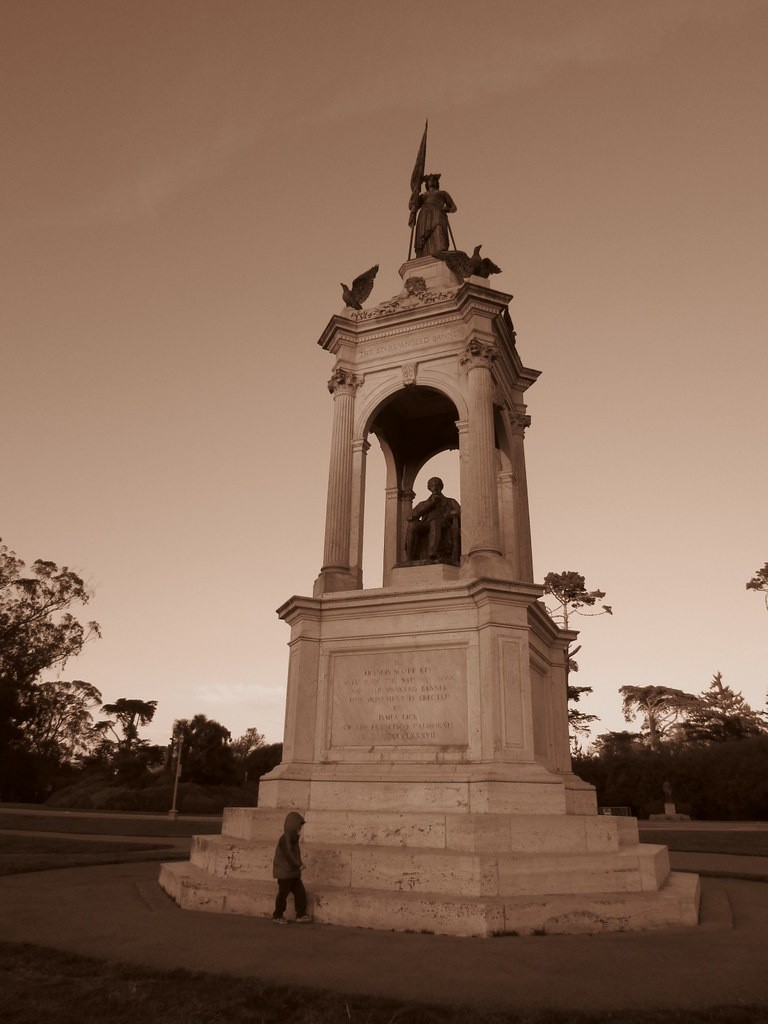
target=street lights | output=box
[167,733,186,821]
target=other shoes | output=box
[273,917,290,924]
[296,915,313,922]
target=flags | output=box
[408,125,429,227]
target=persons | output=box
[414,174,456,259]
[272,812,313,924]
[405,477,462,567]
